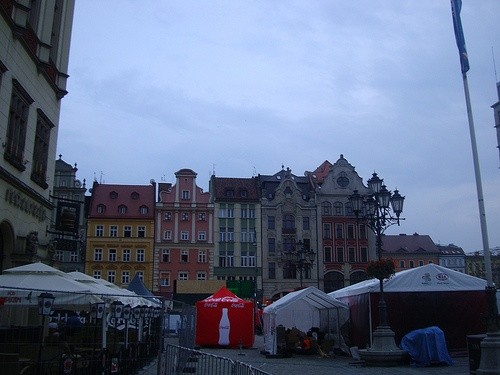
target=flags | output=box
[450,0,470,77]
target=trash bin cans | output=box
[466,333,488,375]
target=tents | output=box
[263,287,350,358]
[327,261,497,349]
[0,260,162,375]
[193,286,255,350]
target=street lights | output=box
[284,239,316,289]
[35,291,56,375]
[348,171,403,327]
[87,295,164,375]
